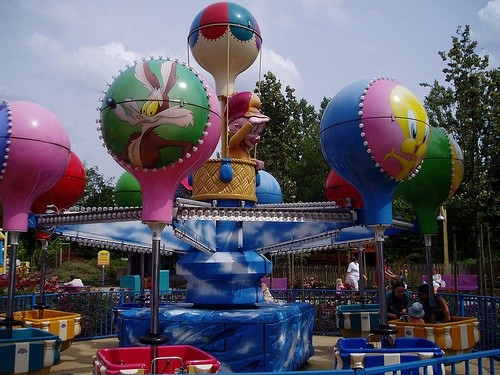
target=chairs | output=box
[68,286,85,292]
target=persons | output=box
[407,302,425,323]
[432,268,442,293]
[106,267,117,287]
[7,256,21,273]
[336,256,368,304]
[262,283,274,301]
[386,281,409,320]
[415,284,453,324]
[400,264,408,277]
[64,274,85,287]
[376,258,398,288]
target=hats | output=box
[408,302,425,317]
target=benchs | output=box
[261,277,287,291]
[421,274,479,291]
